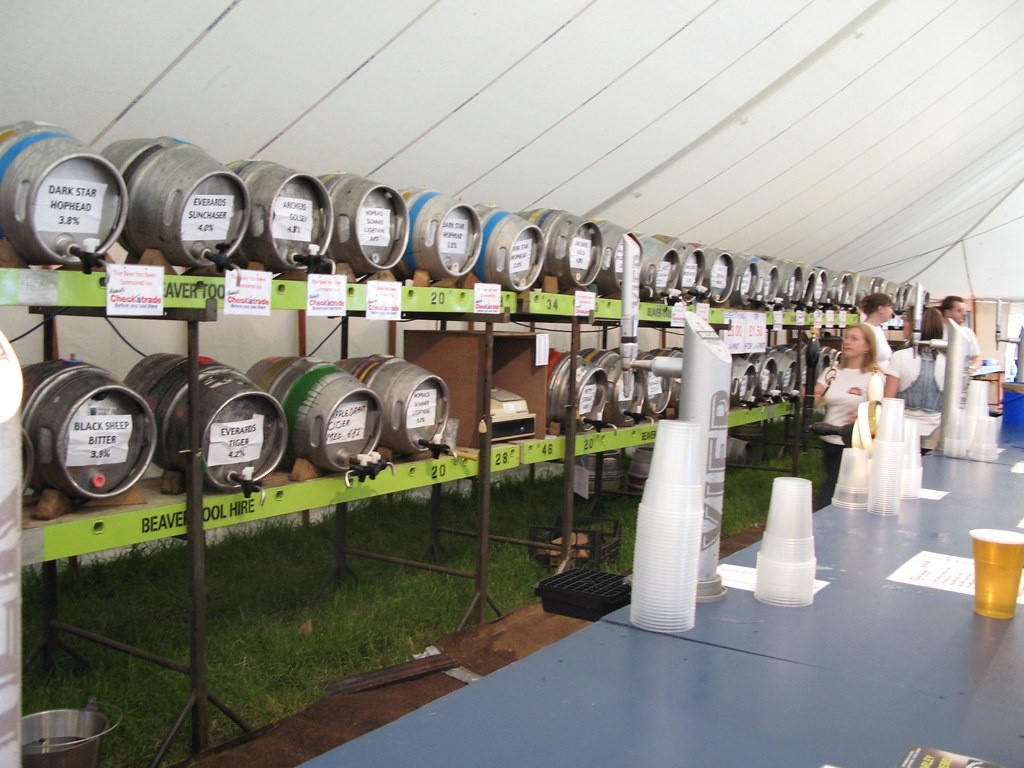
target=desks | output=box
[970,371,1005,406]
[298,415,1024,768]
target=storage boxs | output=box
[406,328,547,450]
[526,514,622,575]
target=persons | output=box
[803,293,983,506]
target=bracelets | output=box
[968,366,978,370]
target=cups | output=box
[830,398,924,516]
[754,477,816,607]
[970,528,1024,620]
[942,380,999,462]
[629,418,708,634]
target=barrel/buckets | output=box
[477,206,931,311]
[0,117,481,497]
[547,346,682,432]
[730,343,842,403]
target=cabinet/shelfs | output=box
[0,263,905,768]
